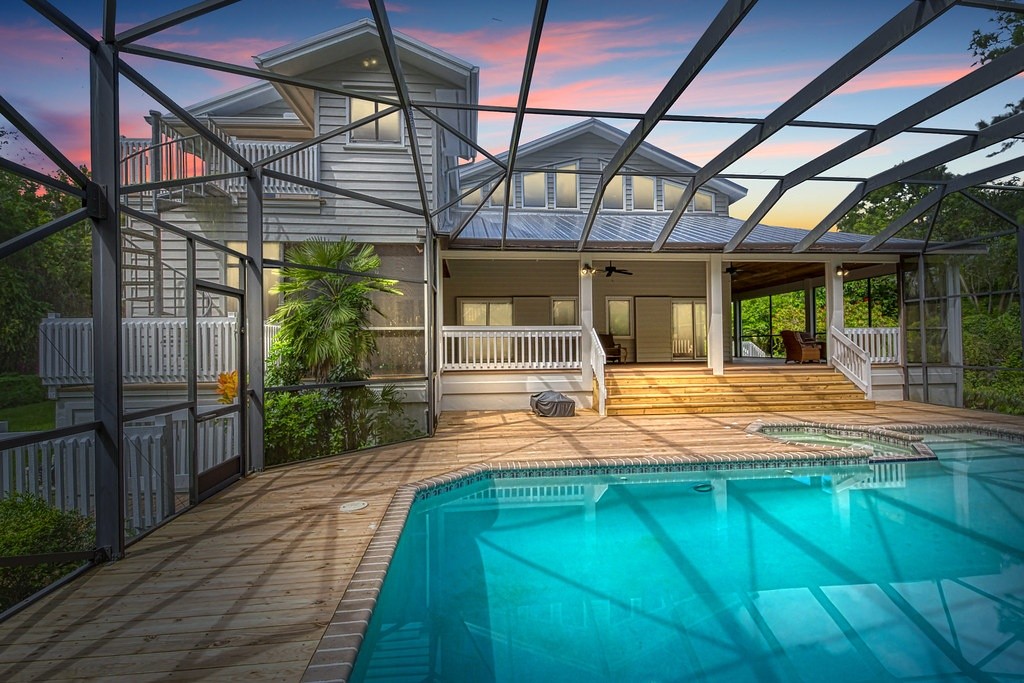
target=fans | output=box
[722,262,757,274]
[596,261,633,277]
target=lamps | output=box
[836,266,849,276]
[582,263,595,274]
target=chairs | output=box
[781,330,821,364]
[597,334,621,363]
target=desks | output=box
[604,348,627,364]
